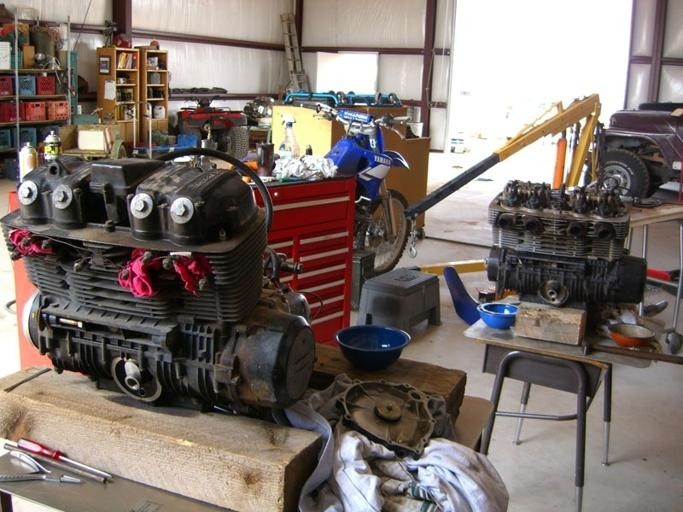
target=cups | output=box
[255,141,277,177]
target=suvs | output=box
[585,101,682,205]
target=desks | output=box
[0,393,495,512]
[628,203,683,329]
[270,104,431,241]
[247,171,357,349]
[463,318,651,512]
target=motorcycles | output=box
[312,103,414,279]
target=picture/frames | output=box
[99,55,111,75]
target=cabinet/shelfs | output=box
[96,47,140,143]
[134,46,169,144]
[0,8,72,158]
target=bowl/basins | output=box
[334,324,411,372]
[475,300,522,331]
[606,323,657,347]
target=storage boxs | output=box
[0,41,13,70]
[1,156,19,180]
[77,124,121,151]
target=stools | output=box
[355,267,443,334]
